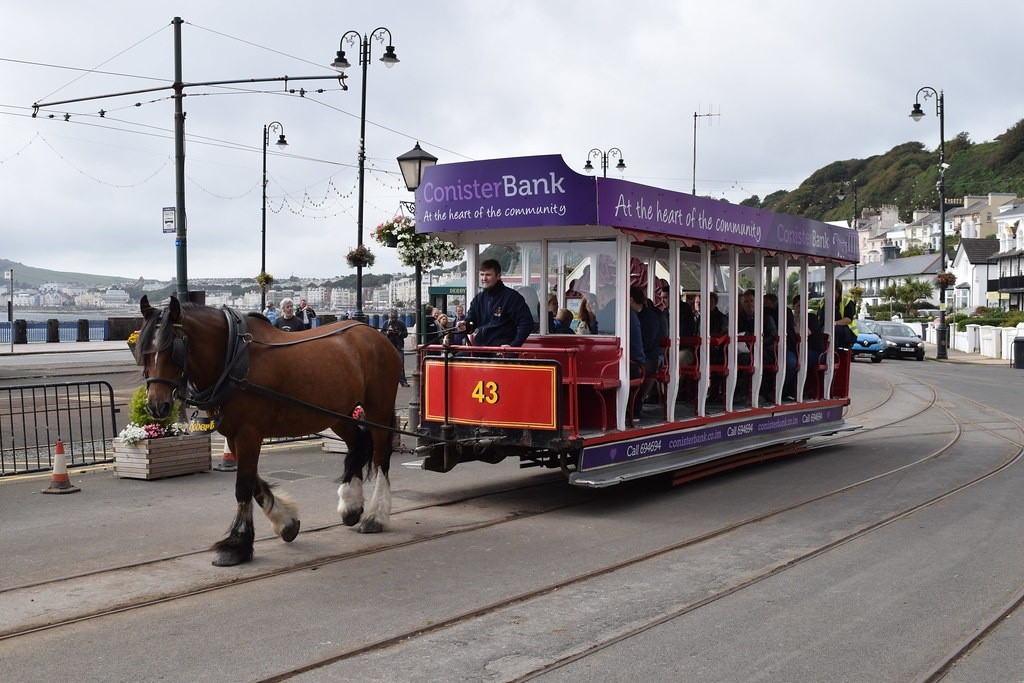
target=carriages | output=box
[136,154,864,567]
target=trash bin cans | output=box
[1010,336,1024,369]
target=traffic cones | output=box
[41,441,81,494]
[213,437,236,472]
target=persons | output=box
[379,309,410,387]
[516,278,858,423]
[452,259,536,361]
[412,305,468,357]
[263,297,316,333]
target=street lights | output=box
[890,297,894,320]
[396,142,438,432]
[584,148,626,178]
[836,177,857,307]
[331,27,401,324]
[261,121,289,313]
[908,86,949,360]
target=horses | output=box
[139,294,404,567]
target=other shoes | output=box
[786,395,795,401]
[401,382,410,387]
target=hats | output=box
[630,287,648,308]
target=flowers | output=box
[120,385,189,445]
[128,331,141,345]
[347,212,464,271]
[936,272,956,285]
[255,271,273,293]
[352,406,364,419]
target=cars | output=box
[851,321,884,363]
[868,321,925,361]
[917,310,940,318]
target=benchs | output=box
[468,331,841,432]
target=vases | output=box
[321,415,401,454]
[112,432,213,480]
[938,282,948,288]
[386,232,397,247]
